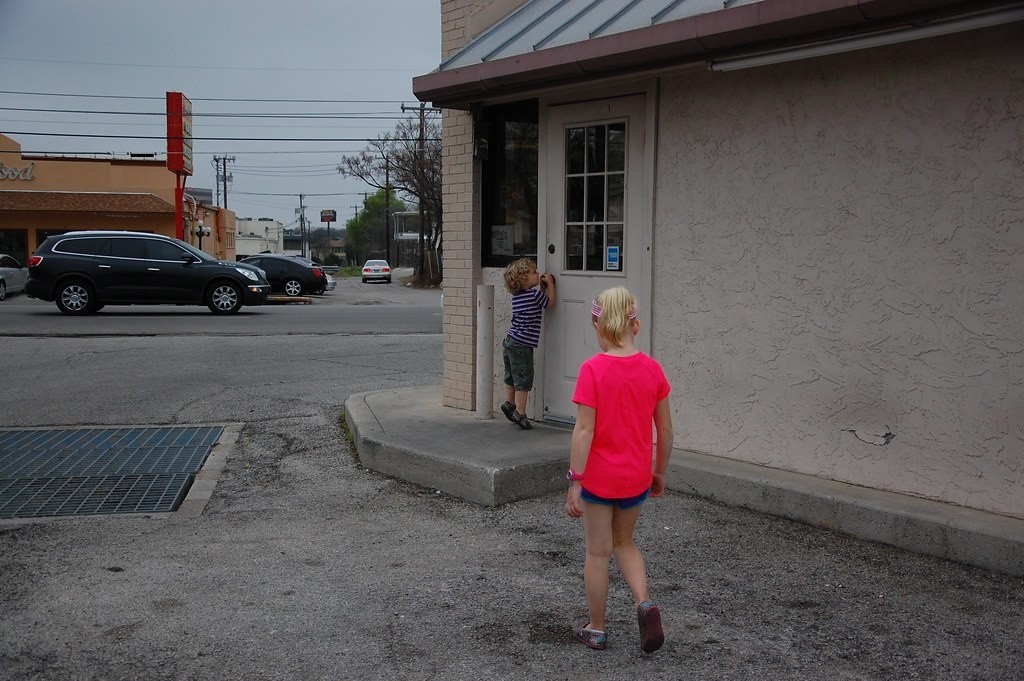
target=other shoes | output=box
[501,401,516,422]
[512,411,532,429]
[573,620,607,650]
[637,599,664,654]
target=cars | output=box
[0,254,29,302]
[310,273,336,294]
[25,230,271,315]
[361,259,392,283]
[237,253,329,297]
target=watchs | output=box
[567,470,584,480]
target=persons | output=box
[501,258,555,429]
[567,287,674,654]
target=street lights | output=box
[193,219,211,250]
